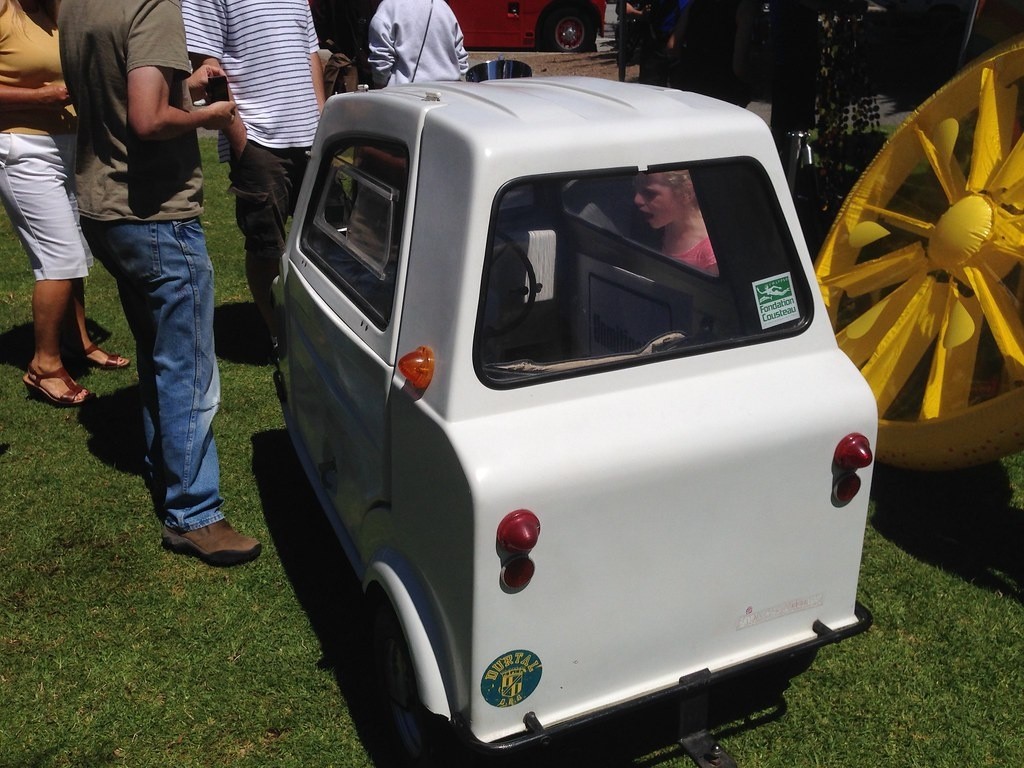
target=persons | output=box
[0,0,130,406]
[617,0,657,85]
[734,0,821,131]
[54,1,263,568]
[634,169,719,278]
[647,0,690,89]
[183,0,349,372]
[367,0,470,82]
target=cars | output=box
[445,0,607,52]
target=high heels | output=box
[23,362,90,407]
[85,343,131,369]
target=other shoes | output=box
[162,516,262,566]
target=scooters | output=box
[268,77,880,768]
